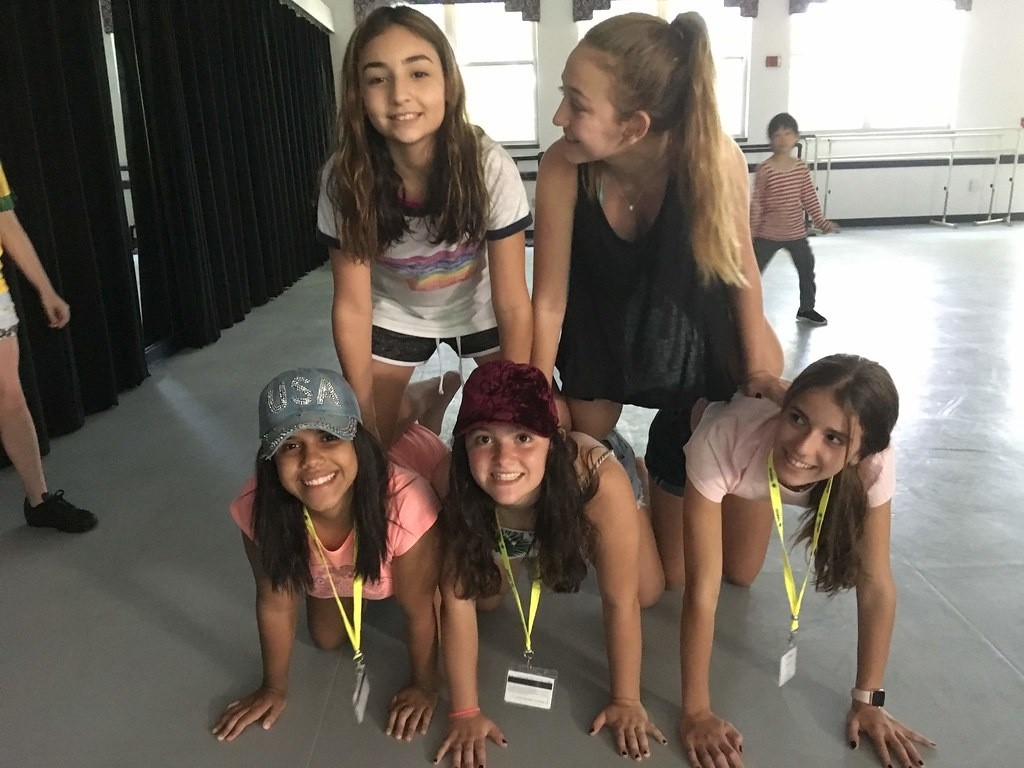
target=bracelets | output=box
[446,706,481,719]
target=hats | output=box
[259,368,363,461]
[452,361,560,439]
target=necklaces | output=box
[609,169,651,212]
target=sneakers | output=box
[24,489,98,534]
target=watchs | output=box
[851,687,885,706]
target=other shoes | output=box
[796,309,827,325]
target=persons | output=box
[430,360,668,768]
[528,11,786,445]
[317,5,573,449]
[212,368,452,741]
[635,354,938,768]
[749,112,841,324]
[0,163,99,535]
